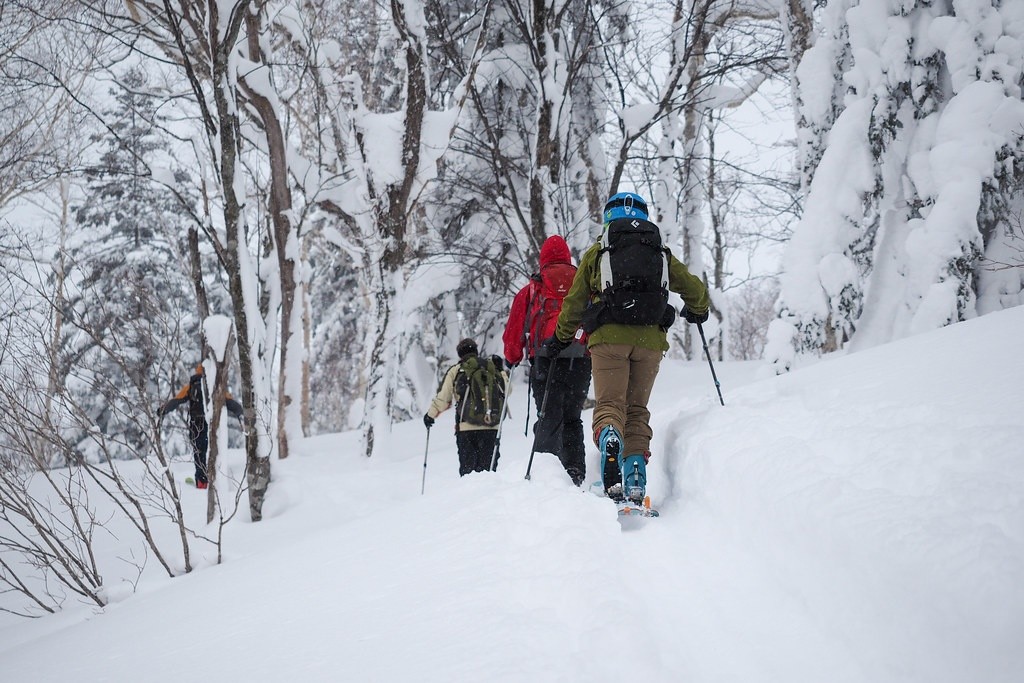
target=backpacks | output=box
[531,262,588,357]
[600,217,670,324]
[455,357,503,426]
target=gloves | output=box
[680,305,709,324]
[546,332,571,357]
[505,359,519,368]
[424,413,434,428]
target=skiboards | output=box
[590,479,657,519]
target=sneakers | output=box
[599,424,623,499]
[624,455,646,501]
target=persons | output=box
[424,338,510,476]
[157,364,243,490]
[544,192,709,504]
[502,235,592,487]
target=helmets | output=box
[603,192,649,223]
